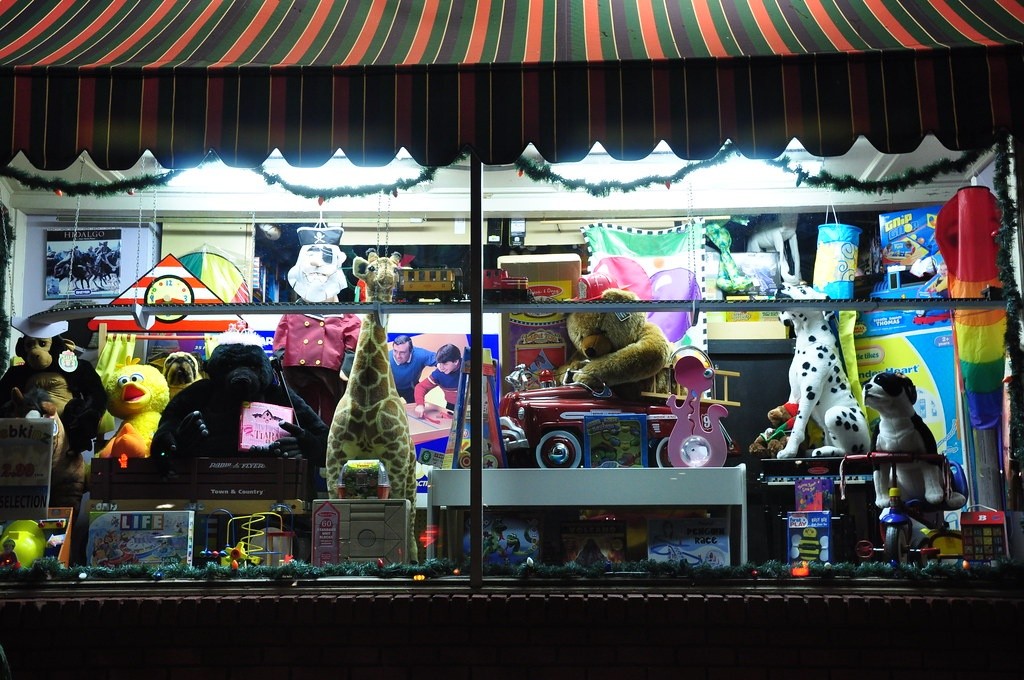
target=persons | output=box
[385,335,437,404]
[47,241,119,273]
[414,344,463,418]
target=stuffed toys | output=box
[0,331,333,512]
[554,287,710,404]
[326,246,419,565]
[861,371,966,508]
[744,283,872,460]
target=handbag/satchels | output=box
[812,202,861,300]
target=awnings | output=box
[0,0,1024,170]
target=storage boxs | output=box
[880,206,945,273]
[704,253,788,340]
[499,252,582,397]
[464,413,731,566]
[84,454,411,569]
[786,511,834,562]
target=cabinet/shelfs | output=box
[427,463,749,565]
